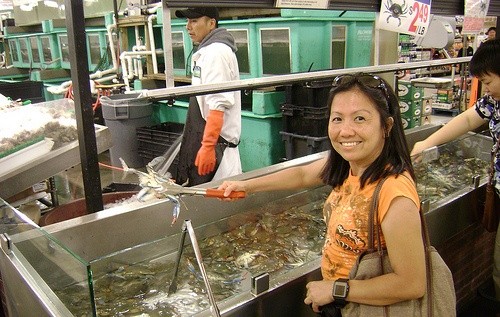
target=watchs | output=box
[333,278,350,307]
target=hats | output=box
[175,6,220,21]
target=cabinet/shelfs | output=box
[1,11,375,114]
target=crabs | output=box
[383,0,408,27]
[119,155,188,228]
[54,150,490,316]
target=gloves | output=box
[194,108,224,176]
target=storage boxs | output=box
[12,0,117,28]
[136,120,186,176]
[0,80,45,105]
[395,33,452,129]
[279,77,336,159]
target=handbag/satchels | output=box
[339,173,456,317]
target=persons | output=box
[410,27,500,303]
[174,4,242,187]
[304,72,428,317]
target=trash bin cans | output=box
[101,94,154,170]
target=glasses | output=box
[332,74,391,108]
